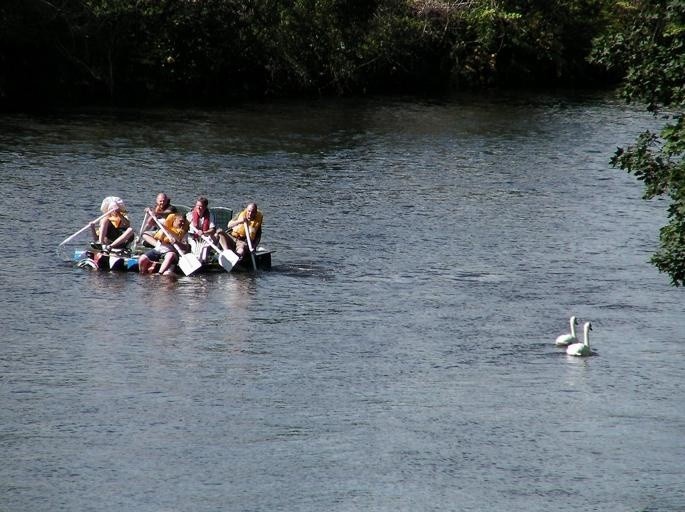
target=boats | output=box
[74,204,277,274]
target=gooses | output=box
[554,316,580,346]
[566,322,594,358]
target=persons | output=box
[185,196,228,263]
[87,202,134,255]
[138,191,186,245]
[138,213,186,279]
[217,202,262,258]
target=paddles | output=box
[189,223,239,272]
[145,209,202,277]
